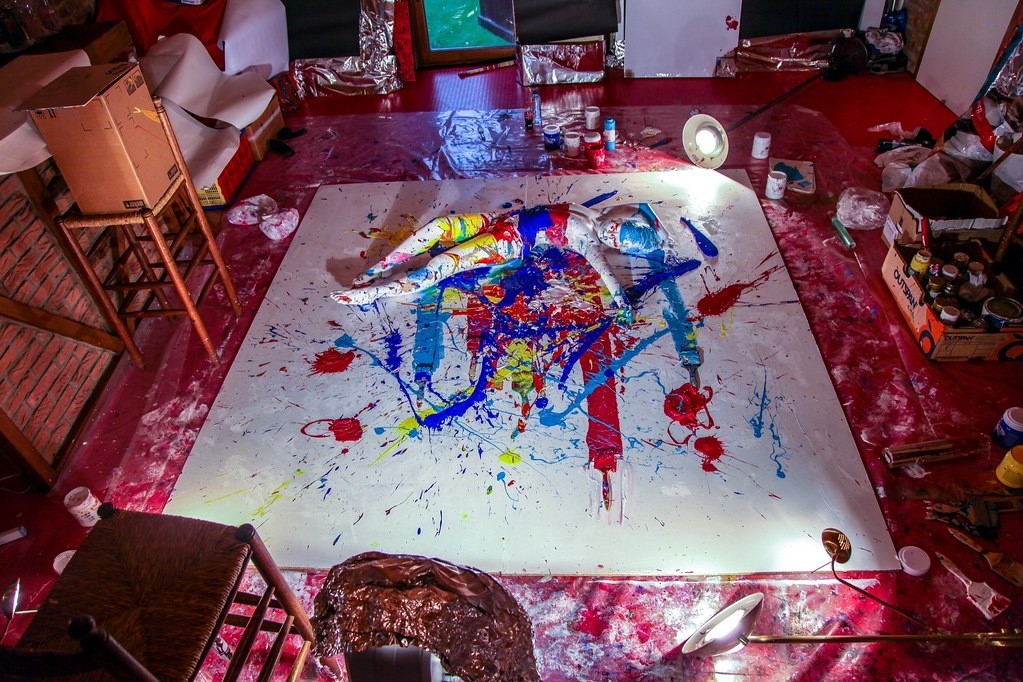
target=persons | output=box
[330,201,677,324]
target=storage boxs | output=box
[880,185,1010,250]
[14,61,182,216]
[186,128,255,211]
[206,95,291,162]
[881,238,1023,363]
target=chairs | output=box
[1,502,318,682]
[53,98,242,373]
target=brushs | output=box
[932,499,998,527]
[935,551,1011,620]
[948,527,1023,587]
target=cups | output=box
[765,171,787,199]
[750,132,772,159]
[584,132,605,169]
[563,132,581,157]
[63,486,103,527]
[543,124,561,150]
[585,105,601,130]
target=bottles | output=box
[910,249,1023,329]
[605,118,615,151]
[993,407,1023,488]
[529,89,542,124]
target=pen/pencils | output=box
[15,610,38,614]
